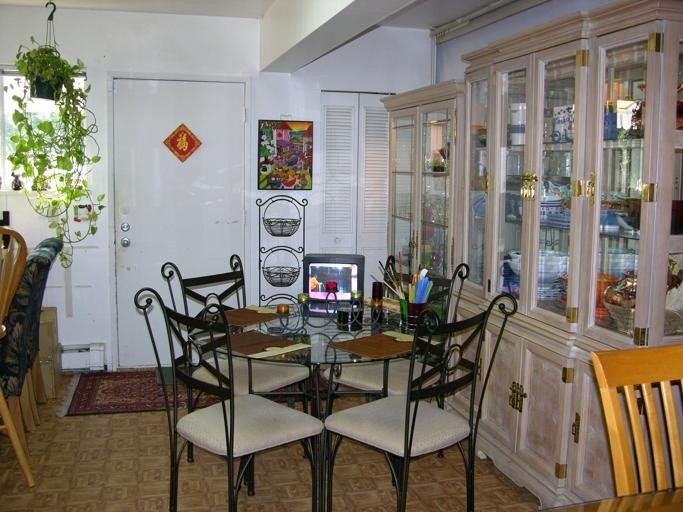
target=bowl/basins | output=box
[509,249,567,284]
[606,248,635,273]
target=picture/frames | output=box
[257,119,314,191]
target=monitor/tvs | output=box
[302,303,364,319]
[302,252,365,305]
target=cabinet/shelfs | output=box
[461,8,589,332]
[383,80,463,281]
[447,280,683,510]
[581,1,683,349]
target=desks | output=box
[187,303,443,489]
[545,483,682,511]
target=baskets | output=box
[262,249,300,286]
[262,199,301,236]
[601,296,683,337]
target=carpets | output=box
[67,364,341,417]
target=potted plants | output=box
[2,36,106,264]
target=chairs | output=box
[590,344,683,495]
[161,252,312,463]
[134,287,325,510]
[0,227,60,489]
[325,257,470,457]
[327,291,518,509]
